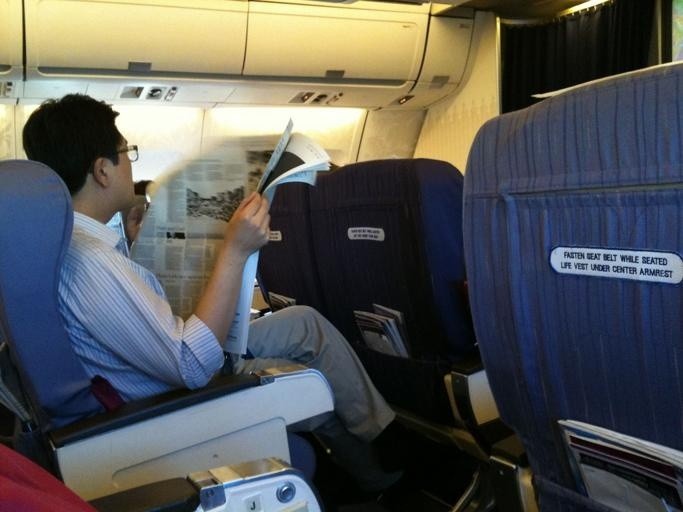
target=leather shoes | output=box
[368,430,459,471]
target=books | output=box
[267,291,296,311]
[1,342,39,434]
[351,301,410,362]
[557,411,683,512]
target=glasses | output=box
[115,144,139,163]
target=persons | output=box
[21,92,411,474]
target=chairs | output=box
[252,161,342,320]
[319,157,507,512]
[1,157,336,501]
[0,440,325,512]
[461,62,683,511]
